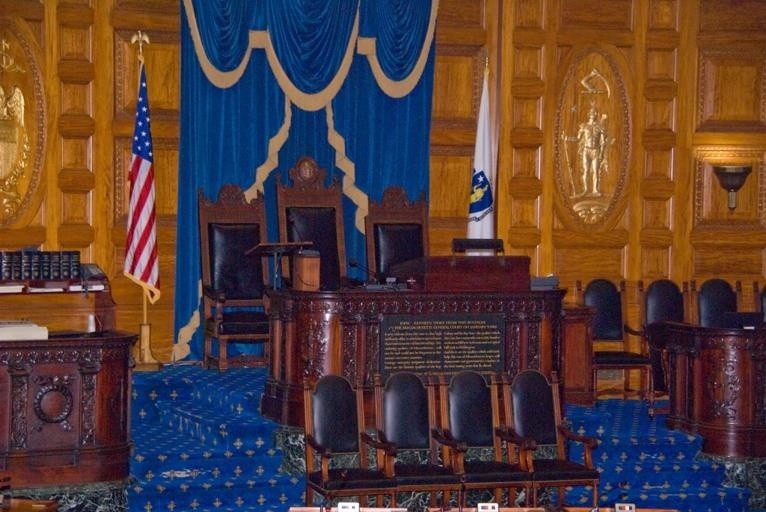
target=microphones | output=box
[286,211,309,250]
[345,255,403,290]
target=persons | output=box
[559,104,608,200]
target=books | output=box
[0,317,48,342]
[87,283,104,290]
[66,284,84,293]
[27,286,63,293]
[0,247,107,282]
[43,280,70,288]
[0,288,22,293]
[0,285,25,288]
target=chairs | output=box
[363,181,429,283]
[275,155,350,284]
[499,369,598,511]
[574,279,766,402]
[437,369,533,512]
[371,369,461,512]
[197,181,275,372]
[305,369,392,509]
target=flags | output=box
[121,60,162,307]
[462,54,496,258]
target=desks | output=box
[657,321,764,458]
[263,281,594,428]
[0,327,141,490]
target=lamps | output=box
[716,163,754,211]
